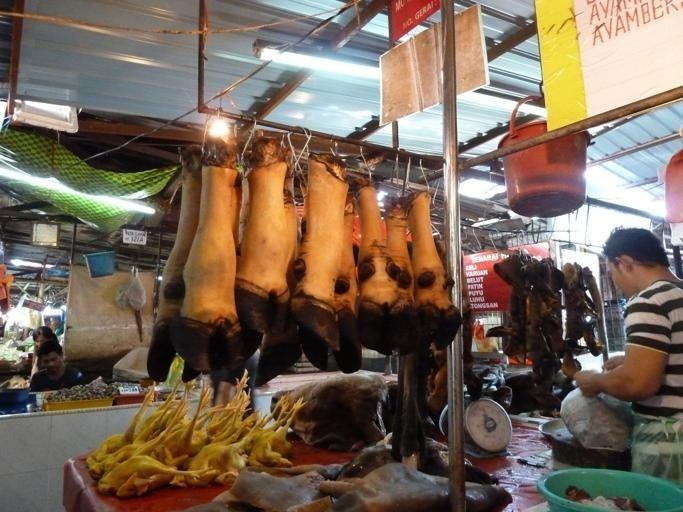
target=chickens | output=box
[84,367,308,497]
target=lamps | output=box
[252,37,381,84]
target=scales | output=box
[439,394,513,460]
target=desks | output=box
[60,413,683,510]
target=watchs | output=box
[210,345,259,426]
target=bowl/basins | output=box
[537,467,683,512]
[0,389,31,405]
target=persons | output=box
[573,226,683,483]
[28,312,89,392]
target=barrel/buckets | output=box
[83,239,117,278]
[494,94,591,219]
